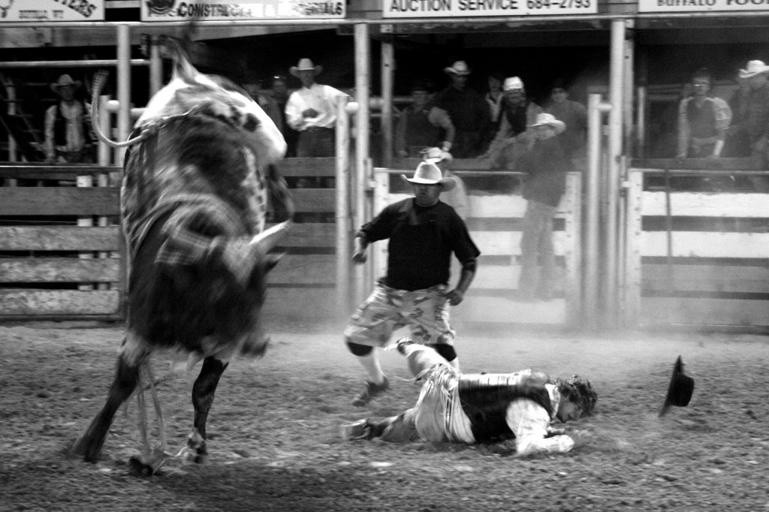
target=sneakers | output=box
[352,377,388,406]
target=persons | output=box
[509,113,569,298]
[479,72,588,170]
[436,61,492,156]
[285,58,354,224]
[332,332,603,456]
[341,158,481,409]
[651,59,769,197]
[394,84,455,156]
[238,76,285,159]
[42,75,108,164]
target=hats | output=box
[402,147,455,191]
[289,58,321,78]
[51,74,81,93]
[532,113,566,136]
[738,59,769,79]
[445,61,470,76]
[659,356,693,416]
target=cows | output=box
[68,33,297,466]
